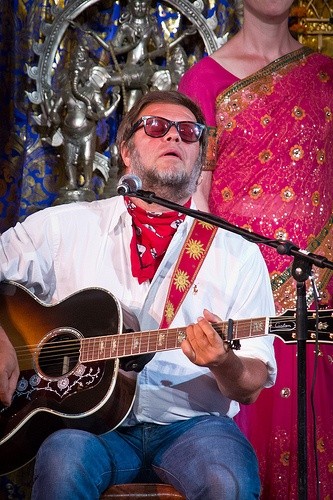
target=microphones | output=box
[115,175,142,196]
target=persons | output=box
[179,0,333,500]
[0,89,277,499]
[113,0,168,112]
[47,43,122,192]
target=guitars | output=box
[0,275,333,478]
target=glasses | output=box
[128,115,206,142]
